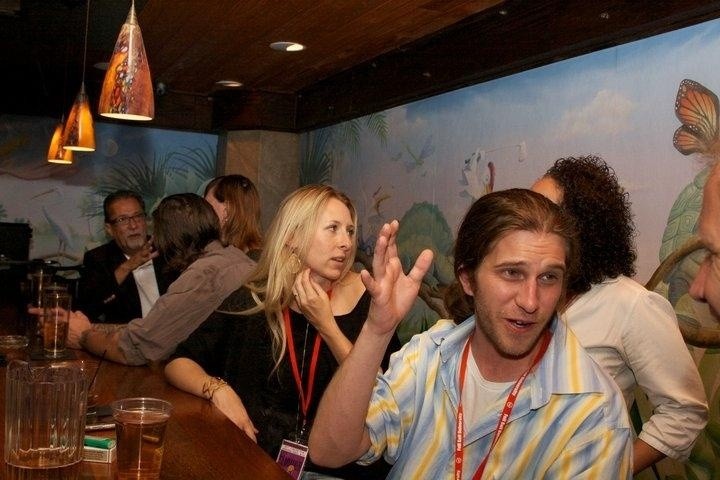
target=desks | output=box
[4,301,293,480]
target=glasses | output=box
[110,212,147,225]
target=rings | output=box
[293,291,301,299]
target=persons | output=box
[79,188,181,323]
[163,185,404,480]
[28,192,260,405]
[530,157,709,475]
[689,131,720,328]
[306,187,634,479]
[202,173,264,261]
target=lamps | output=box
[99,0,154,122]
[47,0,97,165]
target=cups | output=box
[43,285,70,360]
[109,396,174,478]
[6,361,86,478]
[80,359,102,408]
[29,271,58,308]
[0,335,28,364]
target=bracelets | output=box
[198,373,228,403]
